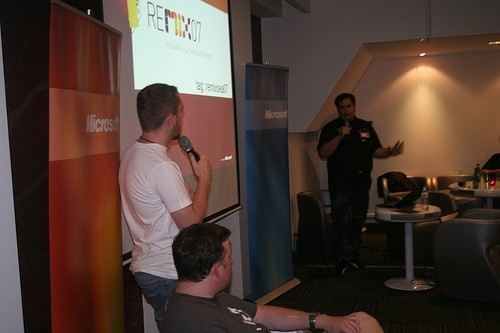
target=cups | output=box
[489,179,495,186]
[459,181,464,186]
[466,181,473,189]
[485,174,490,182]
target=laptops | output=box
[375,188,423,209]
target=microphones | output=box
[344,119,349,141]
[178,135,201,162]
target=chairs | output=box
[294,152,500,303]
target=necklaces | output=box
[139,136,158,143]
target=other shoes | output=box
[336,258,366,274]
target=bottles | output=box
[474,164,481,182]
[473,171,478,189]
[421,186,428,210]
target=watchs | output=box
[308,312,320,329]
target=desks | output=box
[449,179,500,209]
[375,203,441,291]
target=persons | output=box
[118,83,212,332]
[163,222,384,333]
[317,93,406,262]
